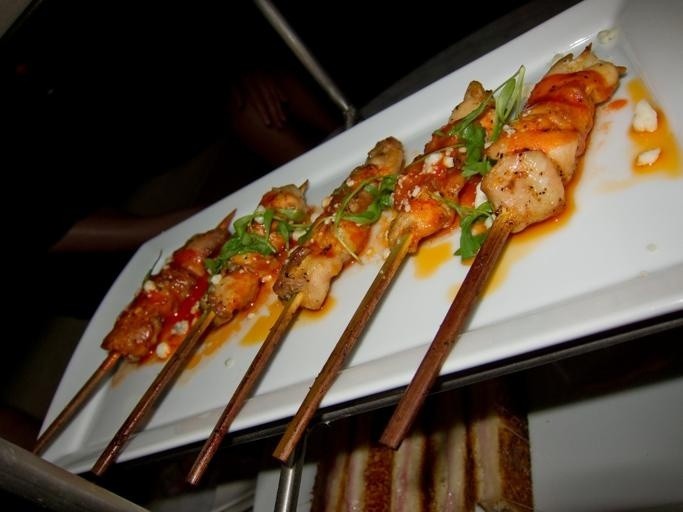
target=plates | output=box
[252,327,683,512]
[36,0,683,476]
